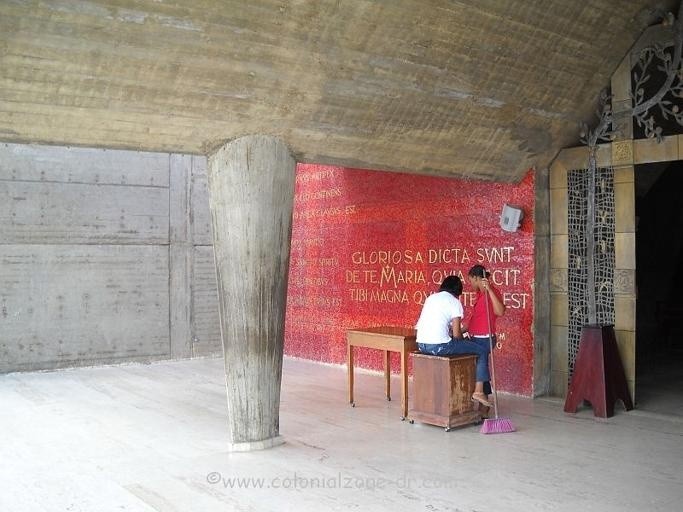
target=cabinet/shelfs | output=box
[409,352,479,432]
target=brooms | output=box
[481,268,517,434]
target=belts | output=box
[471,333,493,338]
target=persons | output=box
[414,276,494,407]
[456,265,505,420]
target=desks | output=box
[345,325,417,417]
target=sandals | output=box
[470,392,494,408]
